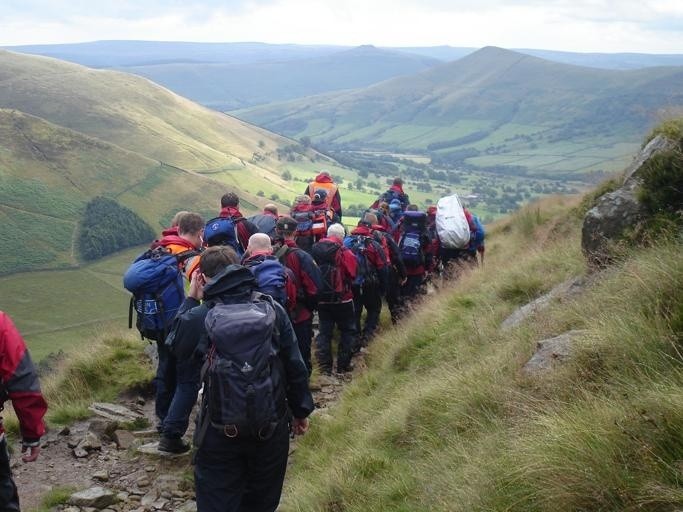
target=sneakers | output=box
[157,439,192,457]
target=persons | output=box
[167,244,315,512]
[154,213,209,455]
[0,309,48,512]
[149,172,484,378]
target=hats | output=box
[392,178,407,185]
